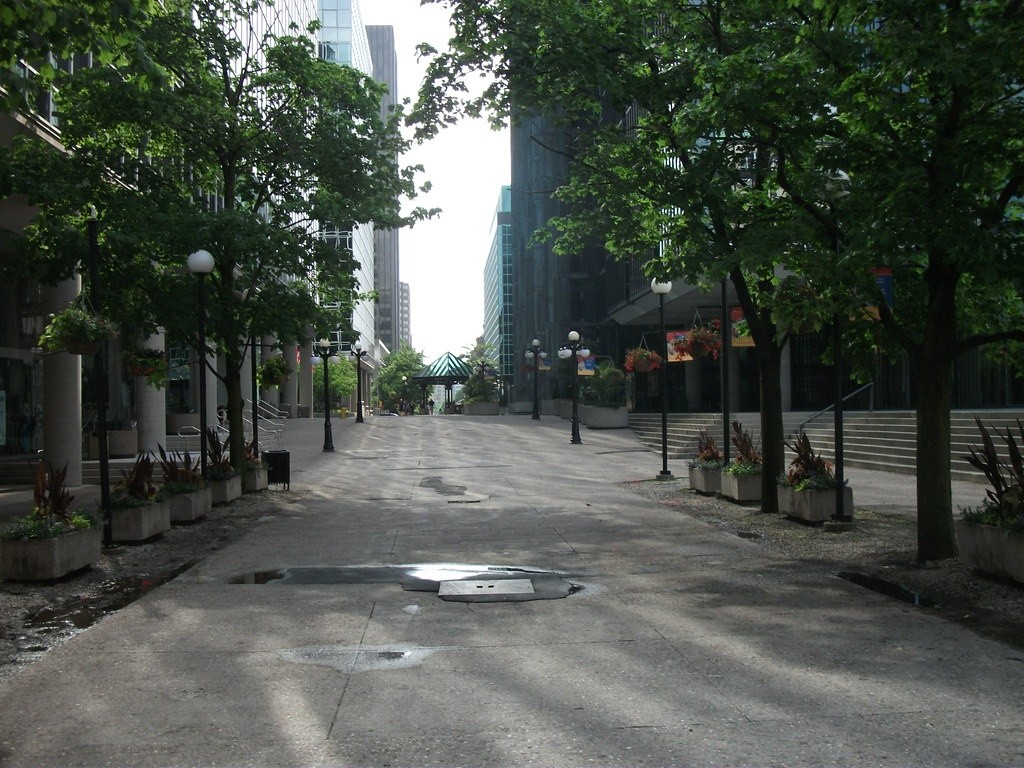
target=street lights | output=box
[311,337,341,453]
[348,341,369,423]
[650,277,675,482]
[525,339,547,420]
[186,249,216,483]
[814,169,853,533]
[242,288,263,461]
[475,357,491,393]
[557,331,590,445]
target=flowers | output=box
[624,347,663,372]
[671,325,721,361]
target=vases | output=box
[689,350,709,359]
[636,363,653,372]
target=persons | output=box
[397,398,408,416]
[439,408,442,415]
[429,399,435,415]
[420,398,426,415]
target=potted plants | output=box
[120,350,170,391]
[0,455,102,579]
[150,443,212,521]
[775,431,853,522]
[96,450,171,540]
[686,429,721,493]
[720,422,761,501]
[462,348,631,428]
[38,306,119,354]
[237,438,269,490]
[254,355,294,390]
[201,427,242,504]
[956,415,1024,584]
